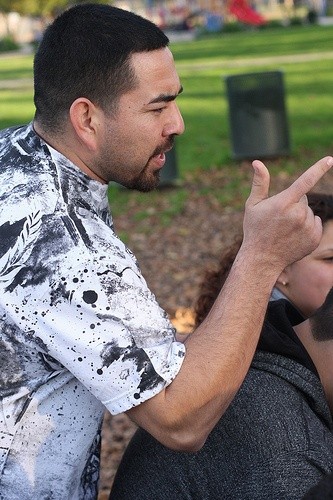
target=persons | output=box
[0,3,333,500]
[110,190,333,500]
[0,0,333,53]
